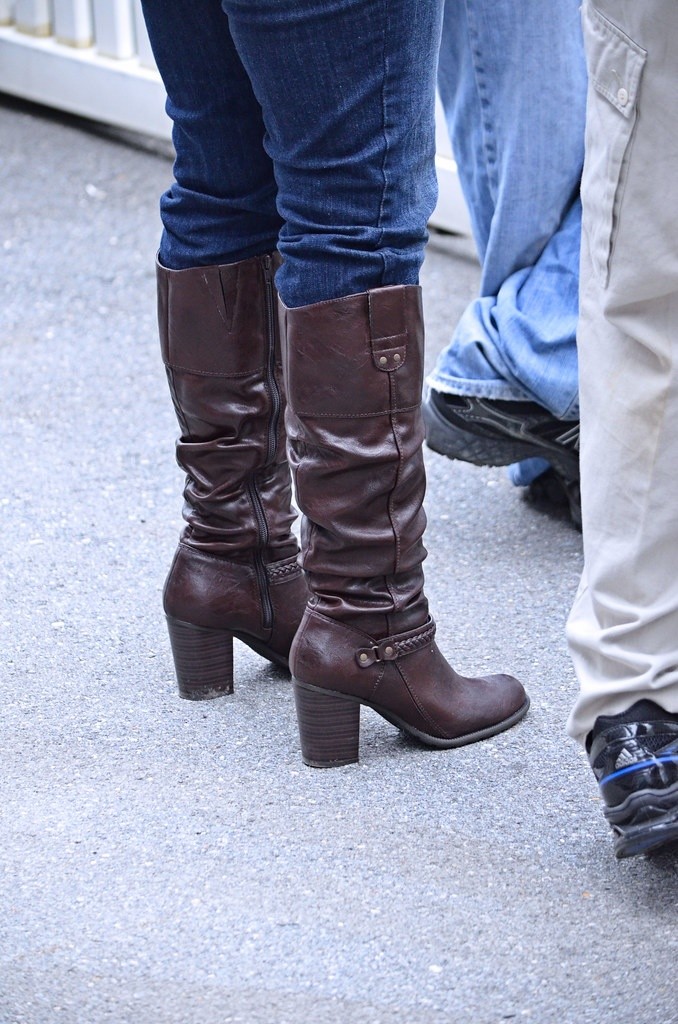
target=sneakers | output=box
[586,699,678,859]
[422,387,582,529]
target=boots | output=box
[277,283,530,768]
[156,244,313,702]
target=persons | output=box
[422,0,588,533]
[142,1,529,767]
[565,1,678,855]
